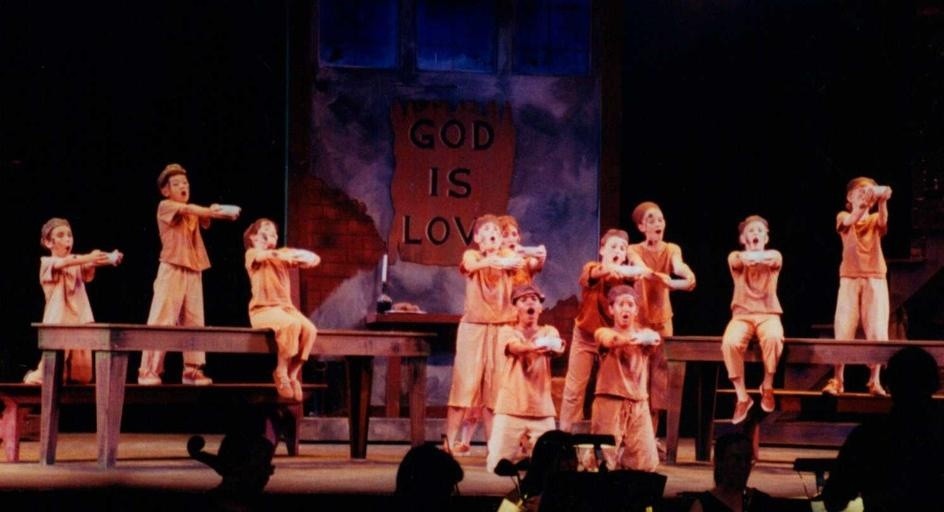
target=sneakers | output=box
[444,444,470,456]
[273,369,294,398]
[656,438,667,461]
[822,379,844,396]
[759,384,775,412]
[866,381,886,397]
[182,370,213,386]
[291,379,303,401]
[731,395,754,425]
[138,368,162,385]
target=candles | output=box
[382,253,388,283]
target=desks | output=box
[29,321,436,471]
[658,336,944,467]
[359,313,463,453]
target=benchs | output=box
[2,383,326,460]
[704,389,942,467]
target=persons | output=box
[821,174,891,398]
[623,200,698,462]
[515,429,585,512]
[19,217,125,388]
[135,160,241,387]
[484,282,567,475]
[198,430,274,511]
[441,213,525,459]
[560,226,645,432]
[457,214,548,458]
[590,283,661,472]
[395,444,464,512]
[720,212,787,423]
[818,344,944,511]
[674,435,786,512]
[241,216,324,402]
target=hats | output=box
[474,214,516,234]
[512,284,545,303]
[610,285,638,304]
[848,177,879,190]
[157,163,187,187]
[40,217,70,240]
[601,201,660,246]
[738,215,769,234]
